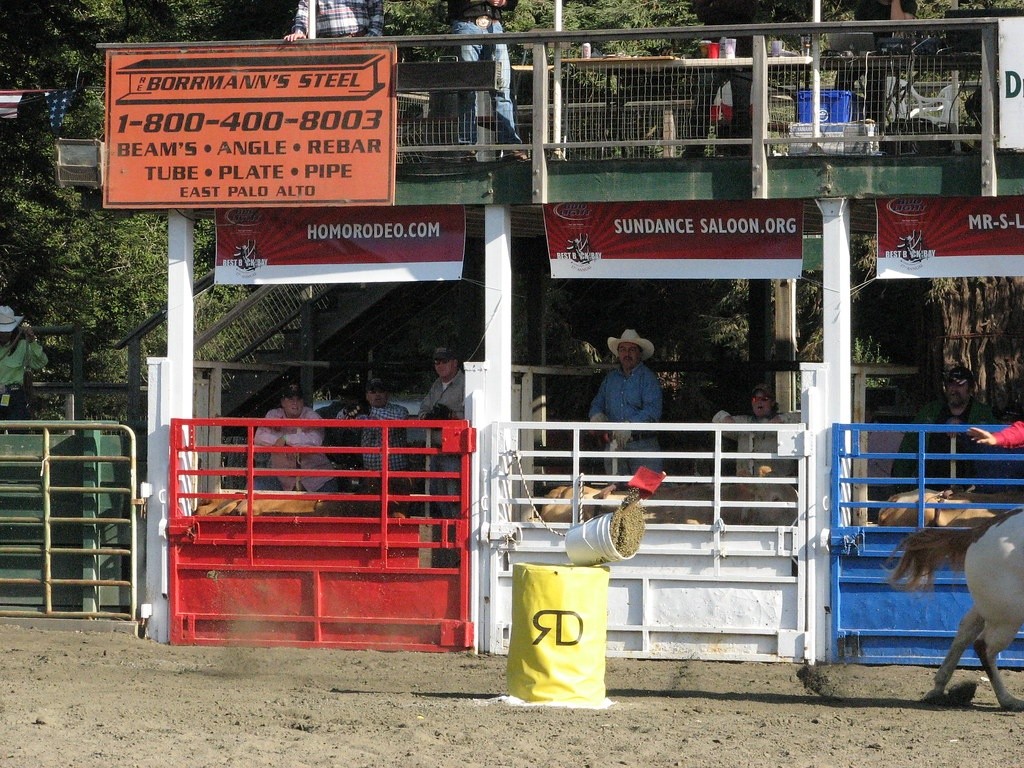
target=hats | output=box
[278,381,306,398]
[608,329,655,362]
[431,347,453,360]
[365,377,387,391]
[944,366,974,388]
[0,304,25,333]
[751,381,775,400]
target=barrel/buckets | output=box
[564,512,636,566]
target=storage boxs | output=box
[789,88,879,154]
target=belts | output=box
[0,383,21,394]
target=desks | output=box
[817,50,999,156]
[574,54,813,158]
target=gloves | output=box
[612,421,633,448]
[589,413,610,423]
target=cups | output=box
[708,43,719,58]
[699,41,711,58]
[771,41,785,57]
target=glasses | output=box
[368,386,385,394]
[433,357,451,366]
[751,395,771,403]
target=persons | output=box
[681,0,757,157]
[712,384,795,477]
[254,346,466,517]
[967,421,1024,449]
[0,306,49,434]
[891,367,992,493]
[284,0,385,42]
[446,0,528,162]
[589,328,664,475]
[836,0,918,120]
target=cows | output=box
[539,470,797,525]
[194,473,414,519]
[874,487,1024,713]
[538,466,798,525]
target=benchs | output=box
[516,98,695,159]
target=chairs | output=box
[885,75,964,156]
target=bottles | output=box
[581,42,592,58]
